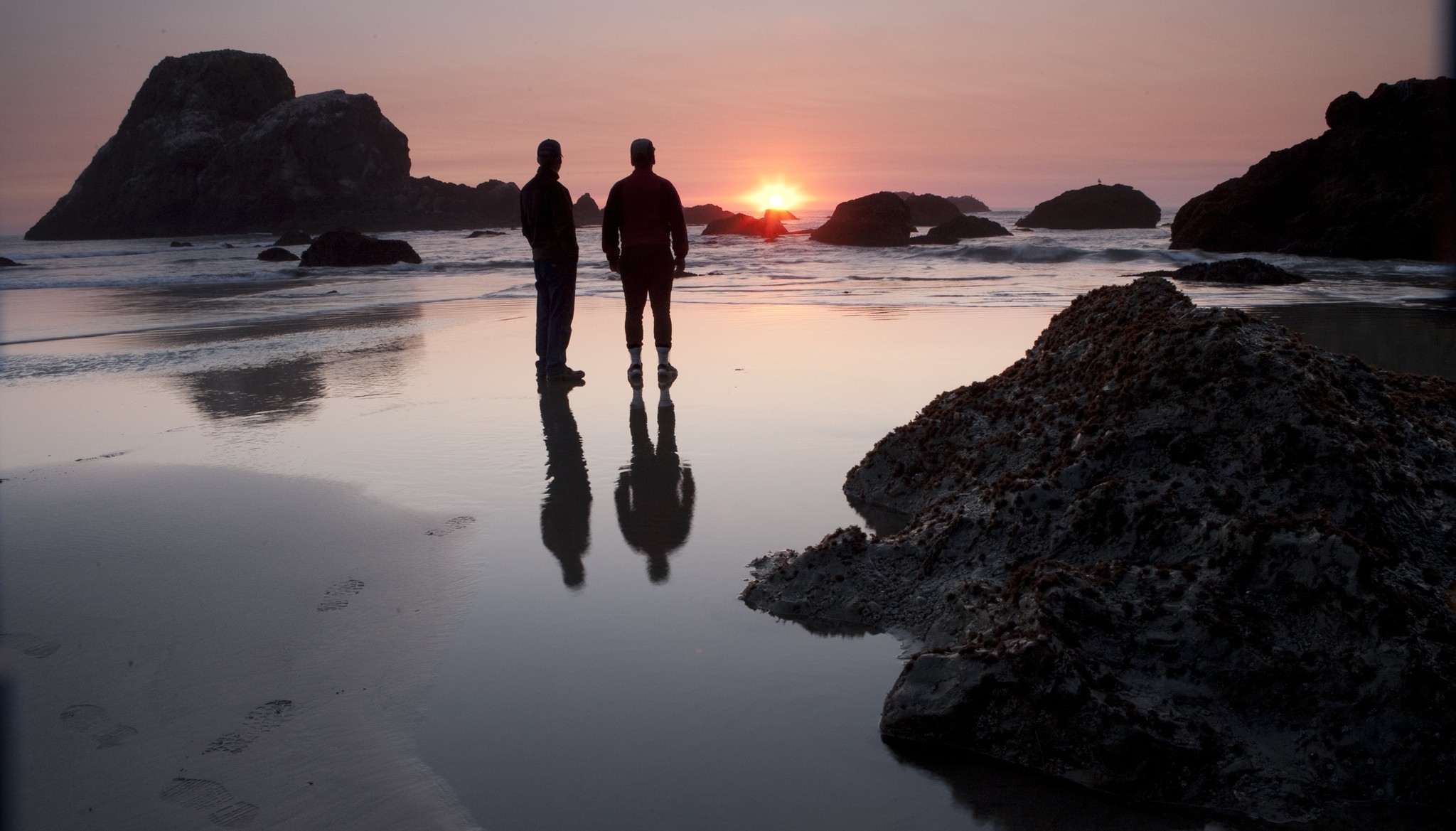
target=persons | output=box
[614,385,696,585]
[534,378,593,587]
[519,139,585,382]
[601,139,689,389]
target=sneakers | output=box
[657,375,677,389]
[627,376,645,389]
[627,363,643,378]
[657,362,678,375]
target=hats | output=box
[538,139,564,162]
[630,137,656,156]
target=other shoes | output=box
[545,362,585,382]
[537,376,548,394]
[545,374,586,394]
[536,359,547,377]
